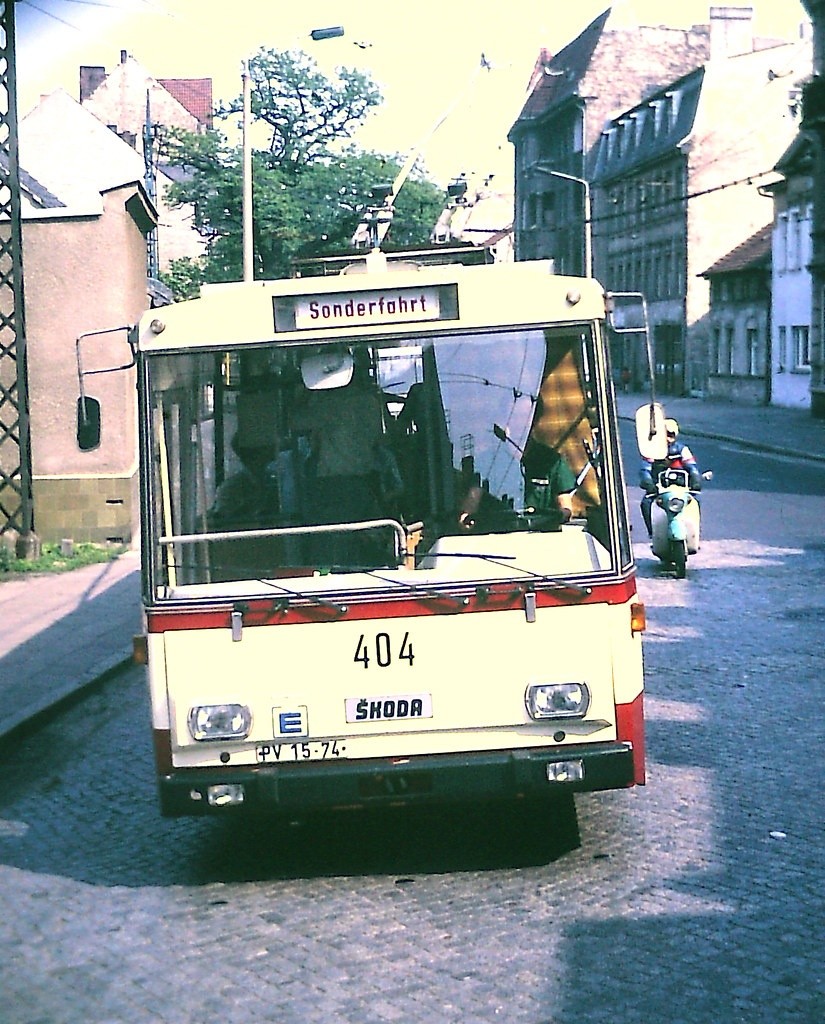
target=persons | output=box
[203,345,426,575]
[639,418,704,551]
[457,383,579,537]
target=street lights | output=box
[534,165,594,280]
[241,25,346,281]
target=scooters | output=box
[638,468,714,581]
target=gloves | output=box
[692,484,702,491]
[646,484,657,493]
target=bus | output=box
[74,237,672,831]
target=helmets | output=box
[666,419,679,441]
